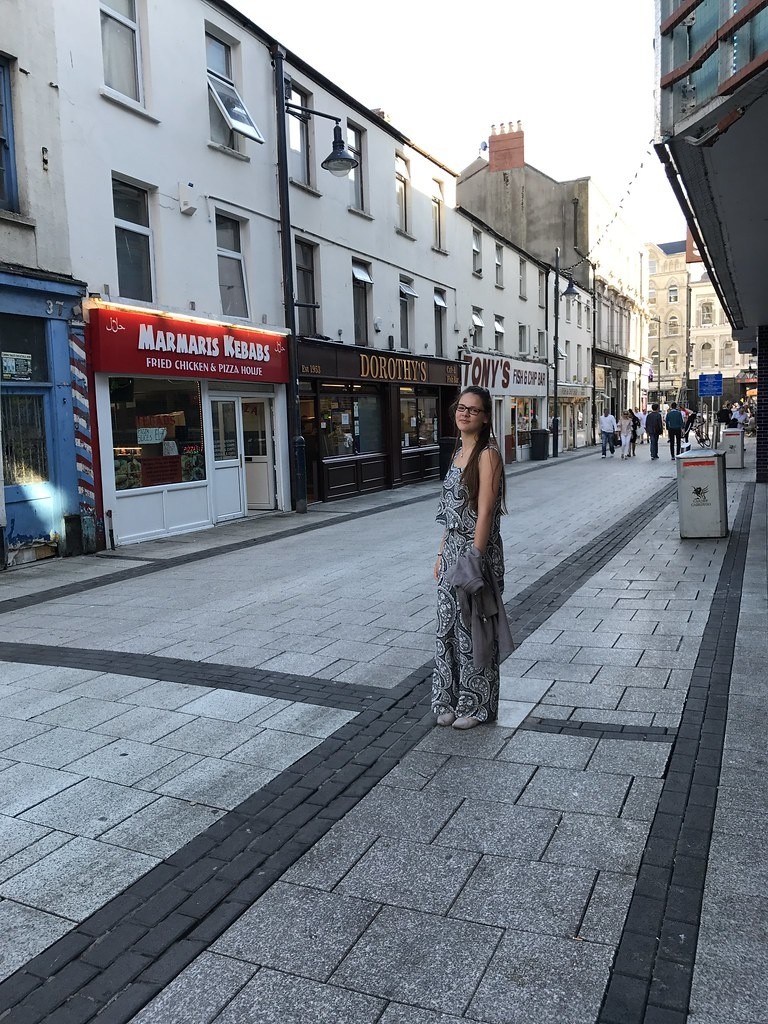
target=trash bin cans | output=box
[718,428,746,469]
[676,447,730,539]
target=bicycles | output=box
[692,417,712,449]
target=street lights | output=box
[551,246,580,458]
[270,50,359,517]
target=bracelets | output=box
[437,553,442,556]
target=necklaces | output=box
[460,442,477,457]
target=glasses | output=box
[454,404,484,415]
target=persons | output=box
[432,386,514,729]
[597,399,756,462]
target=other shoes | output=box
[436,713,480,730]
